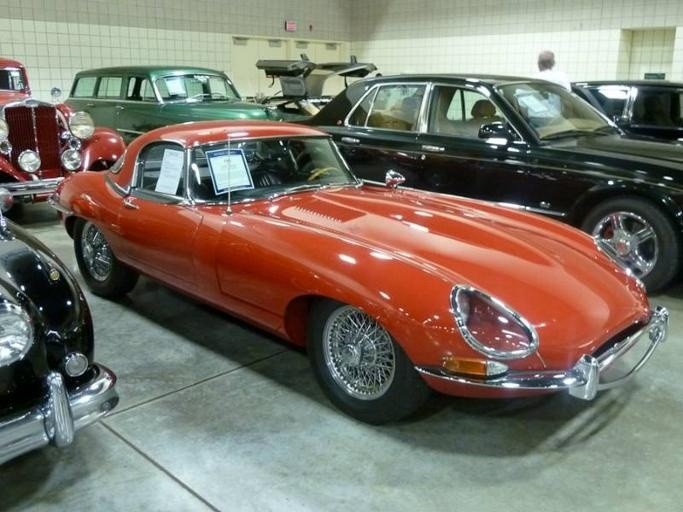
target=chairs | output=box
[250,170,280,186]
[470,99,503,136]
